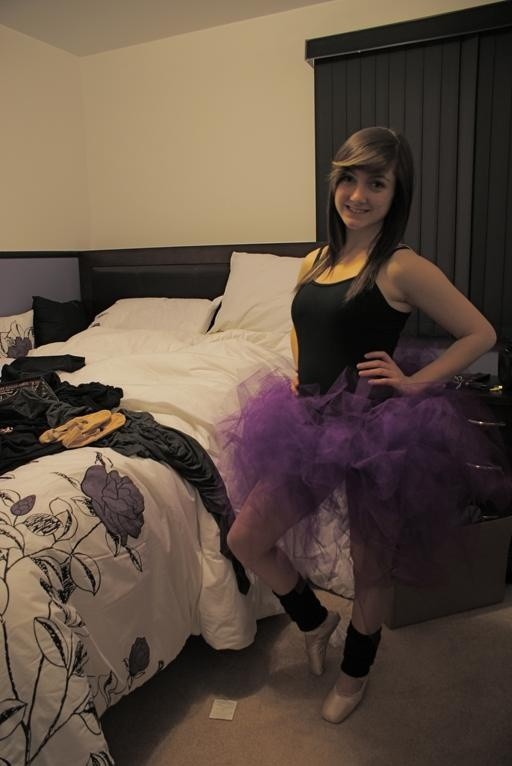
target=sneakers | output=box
[305,611,340,676]
[322,676,368,722]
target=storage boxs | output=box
[382,503,512,631]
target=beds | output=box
[1,238,329,763]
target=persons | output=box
[208,124,499,724]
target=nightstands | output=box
[429,373,512,499]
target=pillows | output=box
[28,288,97,351]
[209,240,314,336]
[1,304,32,361]
[85,291,221,341]
